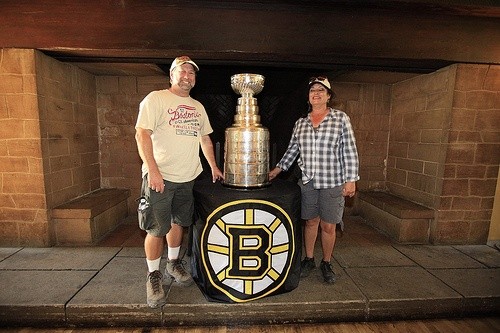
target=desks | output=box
[189,174,303,304]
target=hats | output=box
[169,56,200,72]
[308,77,336,96]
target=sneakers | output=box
[165,248,195,288]
[145,270,167,309]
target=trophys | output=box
[222,73,273,189]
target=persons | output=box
[135,55,225,307]
[268,77,359,284]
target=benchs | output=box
[52,188,132,245]
[360,191,434,246]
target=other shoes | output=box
[320,258,337,285]
[300,256,316,275]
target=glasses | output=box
[309,76,326,82]
